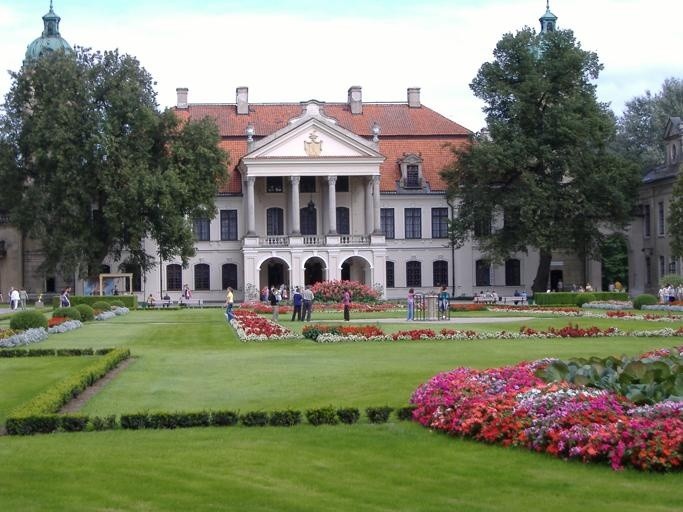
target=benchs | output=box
[147,299,173,307]
[501,296,526,304]
[178,298,202,306]
[472,296,499,305]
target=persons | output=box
[259,283,353,324]
[435,284,450,321]
[543,278,629,294]
[221,285,238,324]
[656,282,682,306]
[3,280,197,315]
[512,289,520,306]
[471,288,500,305]
[519,290,527,306]
[402,287,416,322]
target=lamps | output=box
[306,176,314,214]
